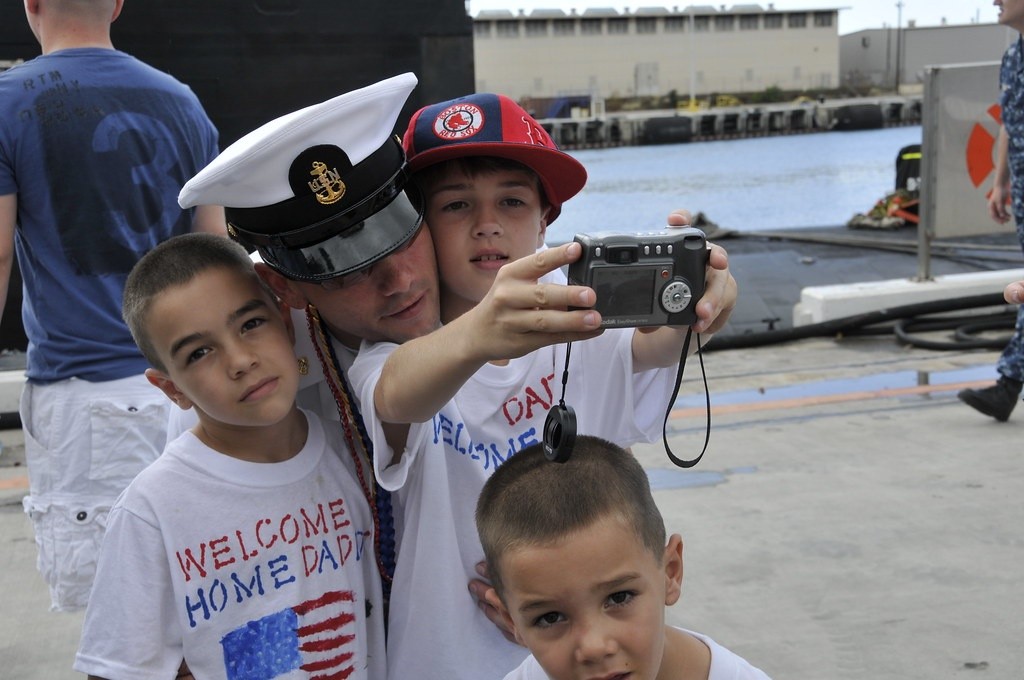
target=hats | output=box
[396,93,587,224]
[179,70,428,279]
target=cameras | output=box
[565,226,708,328]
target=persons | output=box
[346,93,737,680]
[73,234,390,680]
[176,72,445,587]
[473,437,778,680]
[0,0,228,625]
[957,1,1024,421]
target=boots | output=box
[957,376,1023,423]
